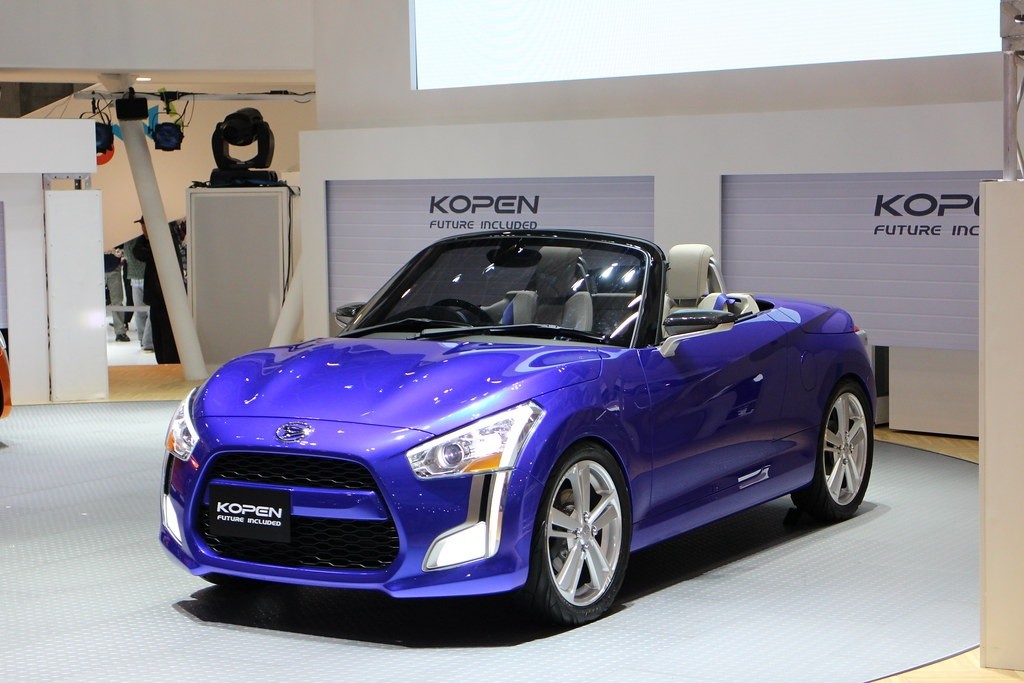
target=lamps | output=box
[76,97,114,165]
[146,94,185,151]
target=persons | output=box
[103,215,186,365]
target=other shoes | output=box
[115,333,130,342]
[109,323,128,331]
[140,344,153,353]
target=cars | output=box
[161,227,878,628]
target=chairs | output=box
[497,243,760,348]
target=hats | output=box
[133,215,144,224]
